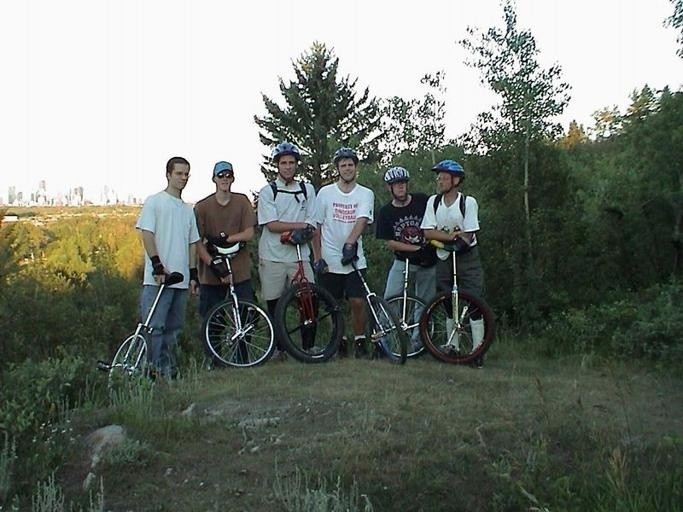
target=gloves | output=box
[209,255,232,278]
[341,241,359,265]
[207,231,233,248]
[415,246,433,262]
[311,258,329,274]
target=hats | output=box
[213,161,232,176]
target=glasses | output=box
[218,173,231,178]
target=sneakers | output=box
[302,345,323,355]
[352,338,369,359]
[338,339,347,358]
[372,347,384,360]
[469,356,484,368]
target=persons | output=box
[418,158,489,368]
[133,156,212,382]
[255,141,322,361]
[189,162,258,369]
[370,166,436,360]
[310,146,377,361]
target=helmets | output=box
[430,160,464,177]
[333,147,358,164]
[383,166,410,184]
[271,142,299,162]
